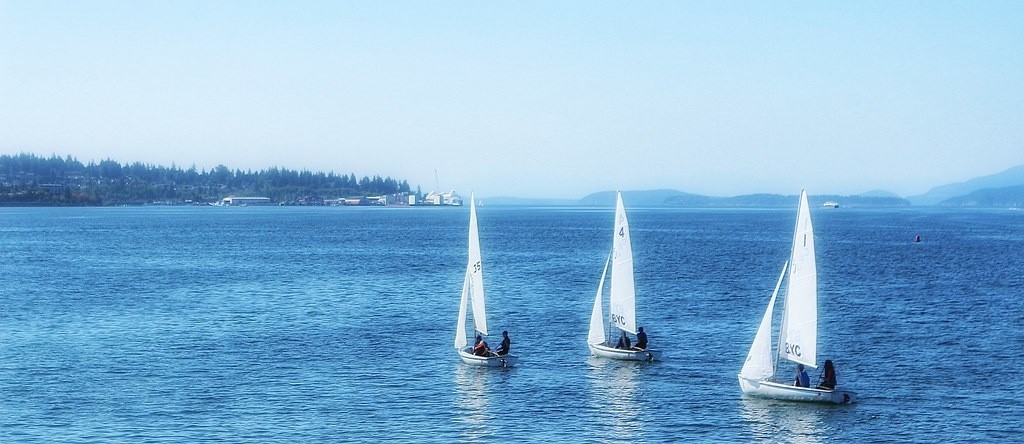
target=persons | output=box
[820,360,837,390]
[614,332,630,349]
[633,327,648,351]
[493,331,510,356]
[792,364,810,388]
[474,335,490,357]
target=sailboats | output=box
[738,187,859,404]
[454,191,520,368]
[583,189,664,362]
